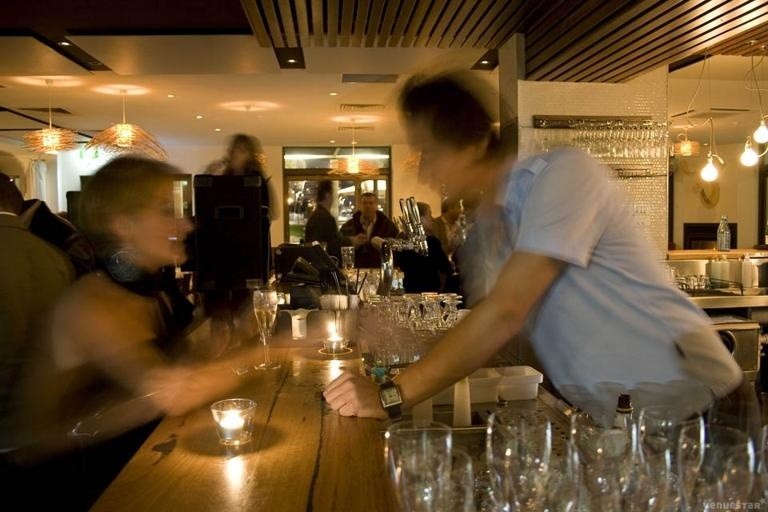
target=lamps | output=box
[685,41,768,183]
[21,79,171,163]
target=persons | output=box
[338,193,399,269]
[305,176,351,268]
[0,171,77,423]
[0,154,315,512]
[191,134,273,324]
[321,65,764,455]
[393,200,460,296]
[429,196,463,257]
[21,197,97,279]
[461,190,483,219]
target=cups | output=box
[382,405,768,512]
[211,396,256,447]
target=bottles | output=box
[710,254,730,288]
[742,253,759,288]
[716,214,731,252]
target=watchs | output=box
[378,380,403,420]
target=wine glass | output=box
[534,118,670,160]
[356,291,464,385]
[252,290,281,372]
[340,247,355,294]
